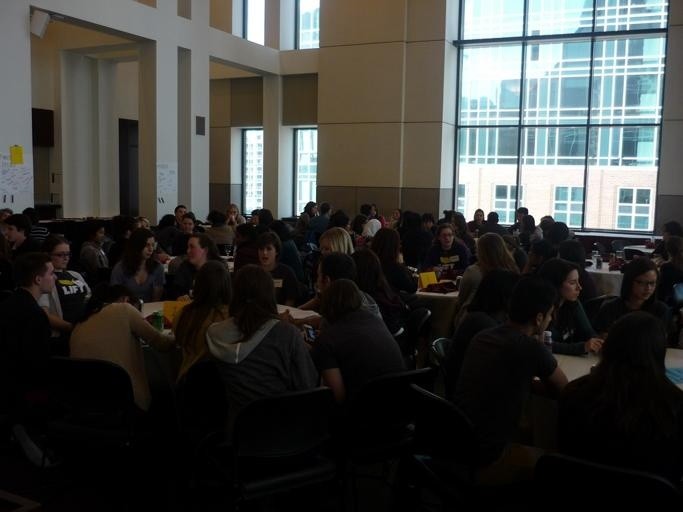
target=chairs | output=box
[582,293,609,309]
[545,453,683,511]
[393,383,501,470]
[610,239,632,254]
[380,366,433,421]
[231,385,339,512]
[592,241,610,257]
[429,337,462,409]
[405,307,434,346]
[39,358,153,479]
[467,286,509,315]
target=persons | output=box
[36,235,94,356]
[251,208,273,230]
[21,207,49,243]
[509,209,571,245]
[451,234,519,337]
[592,258,676,337]
[439,210,455,223]
[557,237,593,306]
[0,252,65,415]
[424,223,471,276]
[125,217,150,238]
[81,219,109,281]
[269,220,305,279]
[316,278,405,402]
[317,251,385,331]
[173,206,188,219]
[203,265,319,442]
[170,233,228,300]
[0,214,40,288]
[552,311,682,491]
[450,212,475,250]
[389,209,402,227]
[208,211,235,262]
[480,211,508,237]
[521,240,556,275]
[372,228,418,299]
[423,213,439,240]
[296,201,317,232]
[653,222,683,255]
[172,211,202,254]
[457,274,571,482]
[656,238,683,305]
[310,202,331,236]
[397,211,432,269]
[329,209,350,228]
[0,208,12,225]
[467,210,486,232]
[298,227,374,316]
[255,232,298,305]
[234,224,255,271]
[67,284,167,410]
[348,205,386,243]
[540,258,603,354]
[225,204,246,225]
[110,229,165,301]
[173,262,231,389]
[156,214,177,240]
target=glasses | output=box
[46,252,72,258]
[634,279,658,286]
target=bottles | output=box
[153,311,163,330]
[594,254,602,270]
[592,242,598,257]
[542,330,552,357]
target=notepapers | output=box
[10,143,25,166]
